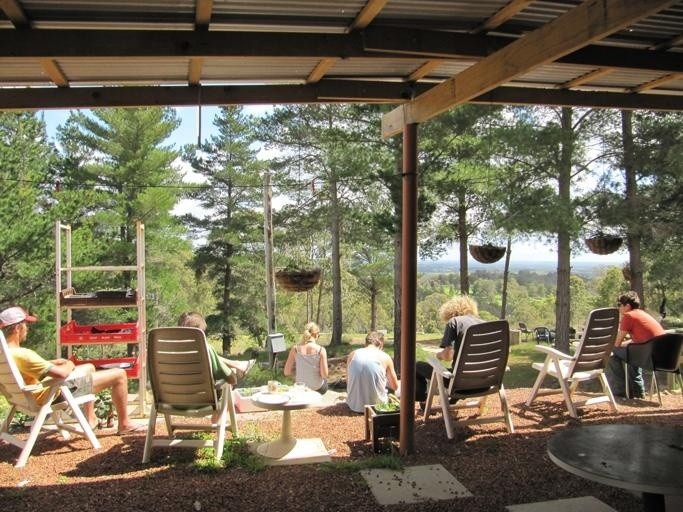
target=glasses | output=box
[617,301,623,308]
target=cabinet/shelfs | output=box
[53,218,148,419]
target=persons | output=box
[414,295,487,414]
[283,321,329,394]
[604,290,665,398]
[163,312,255,423]
[345,331,397,412]
[0,306,137,434]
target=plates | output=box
[253,391,290,405]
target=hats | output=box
[0,306,37,329]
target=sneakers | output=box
[632,393,645,400]
[615,389,632,399]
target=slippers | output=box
[243,358,256,375]
[116,423,151,435]
[91,418,108,435]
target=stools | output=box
[363,401,401,452]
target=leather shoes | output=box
[416,408,432,417]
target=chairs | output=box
[519,322,534,342]
[141,326,238,464]
[624,332,683,407]
[524,307,620,420]
[422,320,514,444]
[0,327,102,468]
[534,327,552,344]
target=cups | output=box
[268,380,279,394]
[293,381,306,393]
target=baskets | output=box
[468,242,505,264]
[278,265,321,293]
[585,233,621,255]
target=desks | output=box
[546,423,683,512]
[250,386,332,466]
